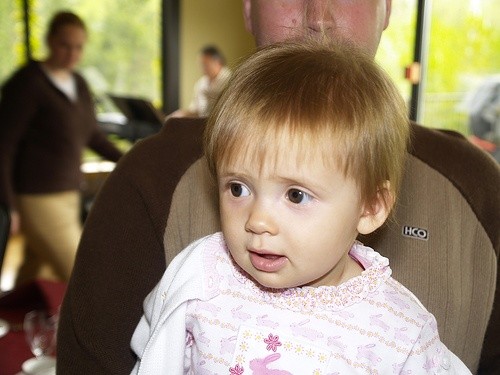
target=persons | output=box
[55,2,499,375]
[165,44,232,119]
[130,27,472,375]
[1,11,134,322]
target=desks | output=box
[97,111,154,138]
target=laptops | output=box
[110,96,166,124]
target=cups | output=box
[21,310,61,374]
